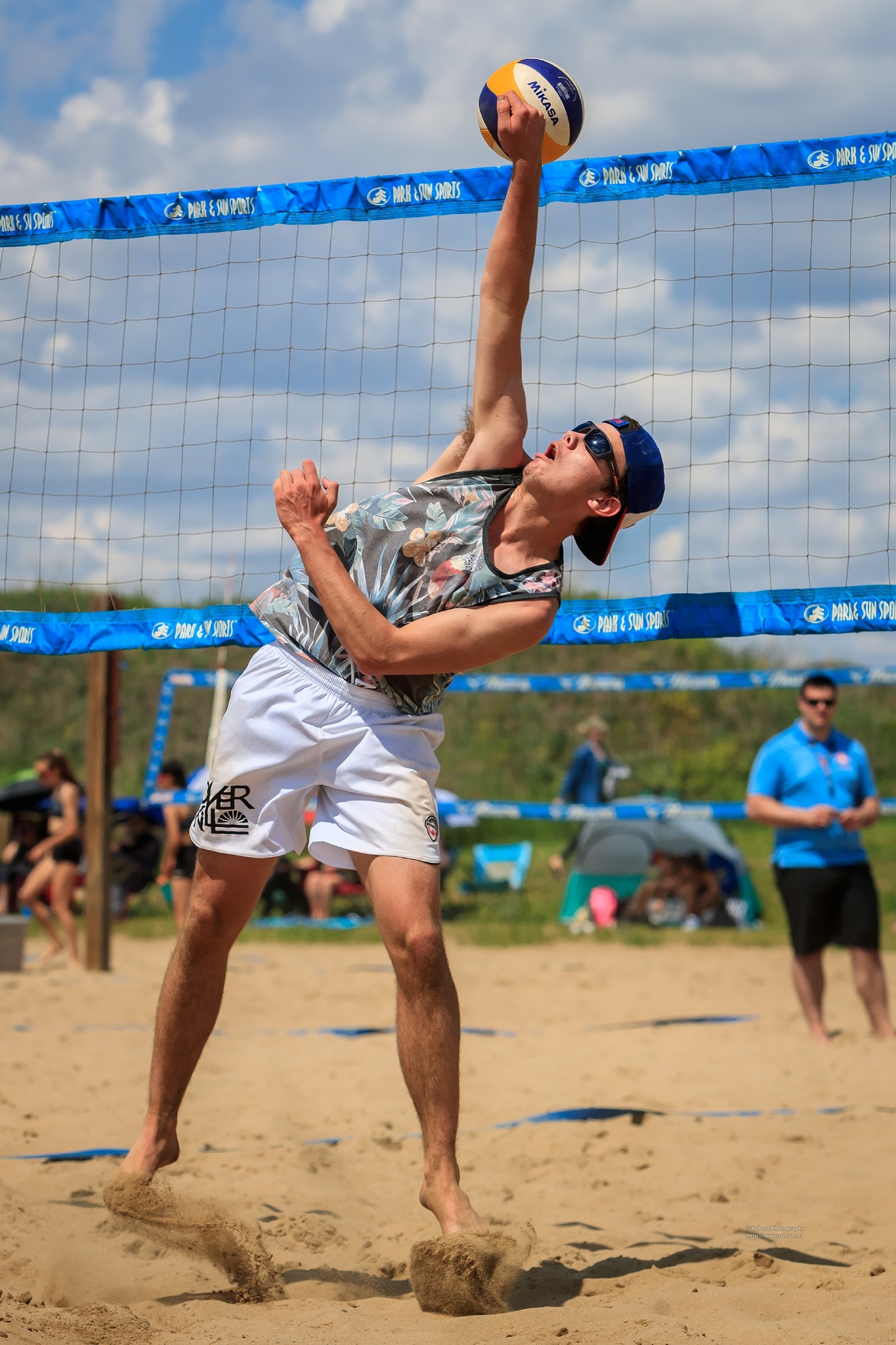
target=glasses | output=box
[803,695,837,708]
[573,420,620,496]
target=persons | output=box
[0,749,456,971]
[746,672,896,1044]
[551,717,728,925]
[104,87,664,1238]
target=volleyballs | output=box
[477,57,584,166]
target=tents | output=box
[558,792,761,930]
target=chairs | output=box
[458,840,537,892]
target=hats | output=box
[574,417,665,567]
